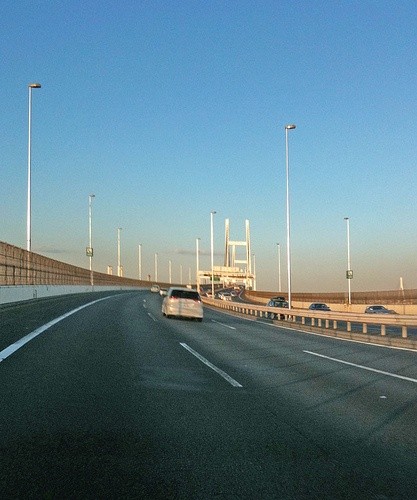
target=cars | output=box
[161,287,204,322]
[158,288,168,296]
[308,303,331,311]
[150,284,161,293]
[205,282,245,301]
[364,305,398,314]
[266,297,294,319]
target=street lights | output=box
[155,252,159,282]
[343,217,351,304]
[210,211,217,298]
[168,259,172,284]
[196,237,202,293]
[138,244,143,280]
[27,82,42,252]
[88,195,96,270]
[284,124,297,319]
[277,242,282,292]
[117,226,123,278]
